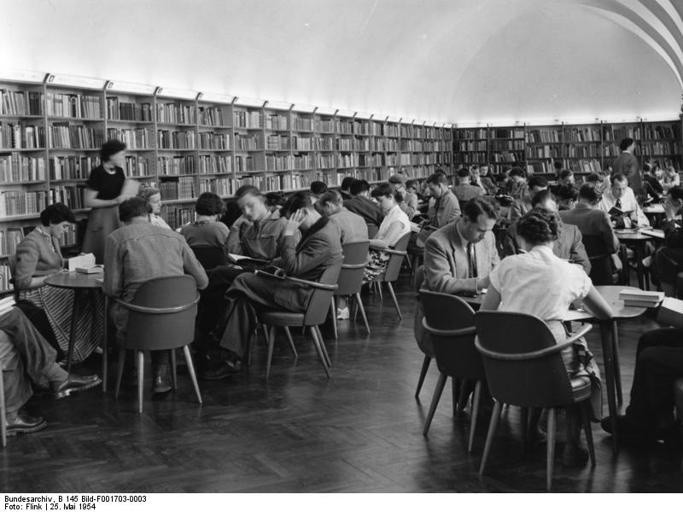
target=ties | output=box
[466,240,476,278]
[615,199,621,208]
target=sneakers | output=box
[330,306,350,320]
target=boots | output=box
[153,365,172,394]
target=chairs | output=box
[112,180,682,492]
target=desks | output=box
[44,266,110,391]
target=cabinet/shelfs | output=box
[0,75,280,294]
[282,109,682,180]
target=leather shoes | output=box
[201,359,245,380]
[49,371,103,399]
[5,414,47,436]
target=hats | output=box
[99,139,126,155]
